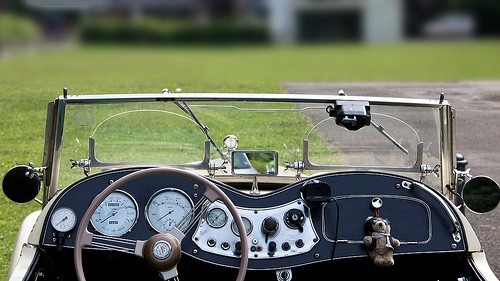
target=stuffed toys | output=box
[363,216,401,266]
